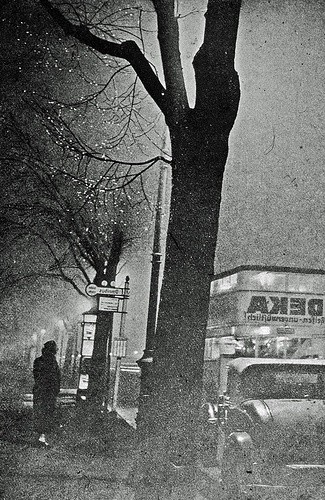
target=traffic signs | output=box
[86,283,123,296]
[97,297,122,312]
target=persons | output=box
[33,340,62,448]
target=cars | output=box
[199,355,325,500]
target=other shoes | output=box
[38,435,51,449]
